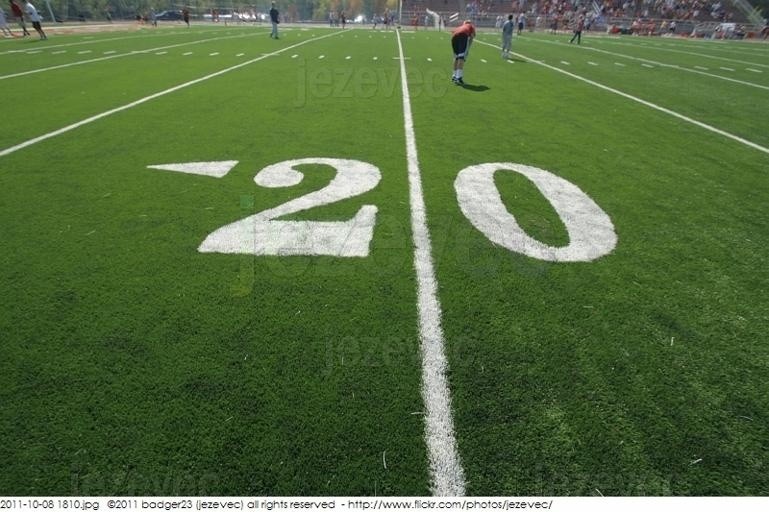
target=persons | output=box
[8,0,31,39]
[466,1,768,41]
[150,7,158,27]
[268,1,281,40]
[326,6,431,34]
[22,0,48,41]
[179,5,190,27]
[211,7,219,23]
[568,15,584,46]
[501,14,513,59]
[0,8,15,38]
[449,19,477,86]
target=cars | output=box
[715,23,746,36]
[154,10,184,20]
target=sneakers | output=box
[452,76,464,87]
[500,51,509,59]
